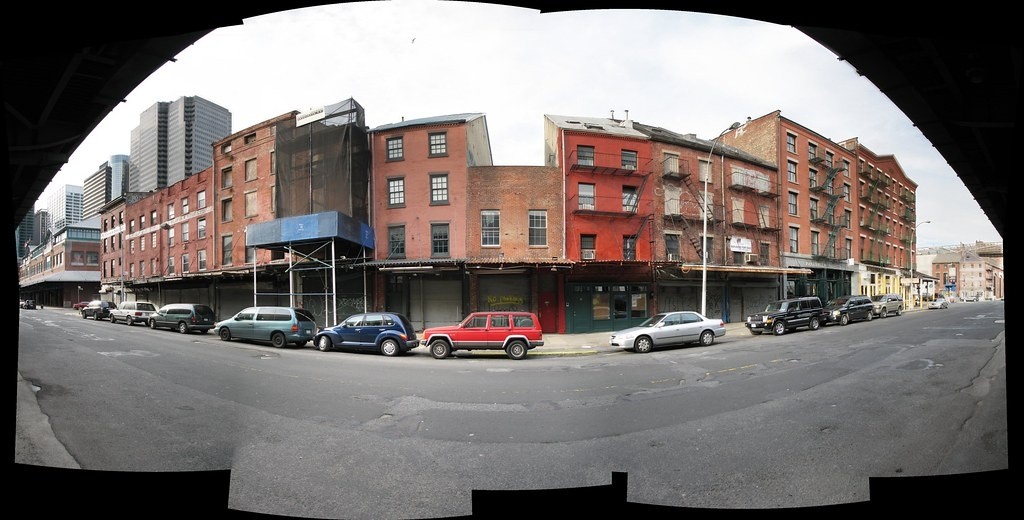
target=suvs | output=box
[421,311,544,361]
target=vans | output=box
[147,304,216,334]
[213,307,317,349]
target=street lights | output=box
[95,209,124,303]
[700,122,742,314]
[909,221,931,310]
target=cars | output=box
[819,294,875,327]
[20,300,36,310]
[109,301,156,326]
[928,298,949,309]
[870,293,904,319]
[312,312,419,357]
[745,297,828,335]
[609,311,727,354]
[73,302,89,310]
[80,300,116,322]
